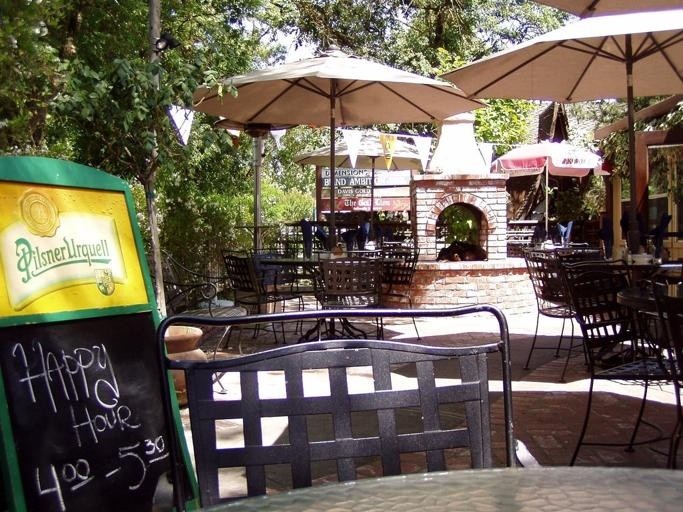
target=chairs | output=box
[220,241,421,347]
[146,251,251,395]
[517,245,682,467]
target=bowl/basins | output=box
[630,254,652,263]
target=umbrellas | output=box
[184,45,488,254]
[528,0,683,34]
[489,139,612,241]
[438,9,683,241]
[293,127,428,240]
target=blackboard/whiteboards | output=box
[0,304,199,512]
